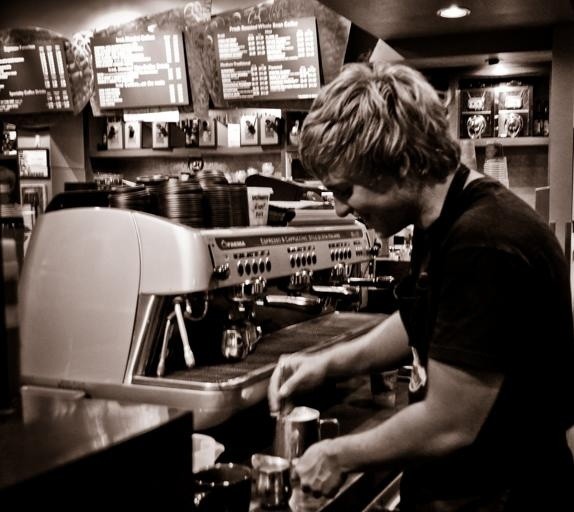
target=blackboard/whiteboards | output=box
[89,32,190,111]
[216,17,323,103]
[0,40,75,115]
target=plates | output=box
[114,171,249,228]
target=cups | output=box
[250,454,293,506]
[369,365,399,404]
[483,143,510,190]
[195,463,252,512]
[273,403,342,472]
[225,162,283,183]
[460,139,477,169]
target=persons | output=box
[263,58,574,511]
